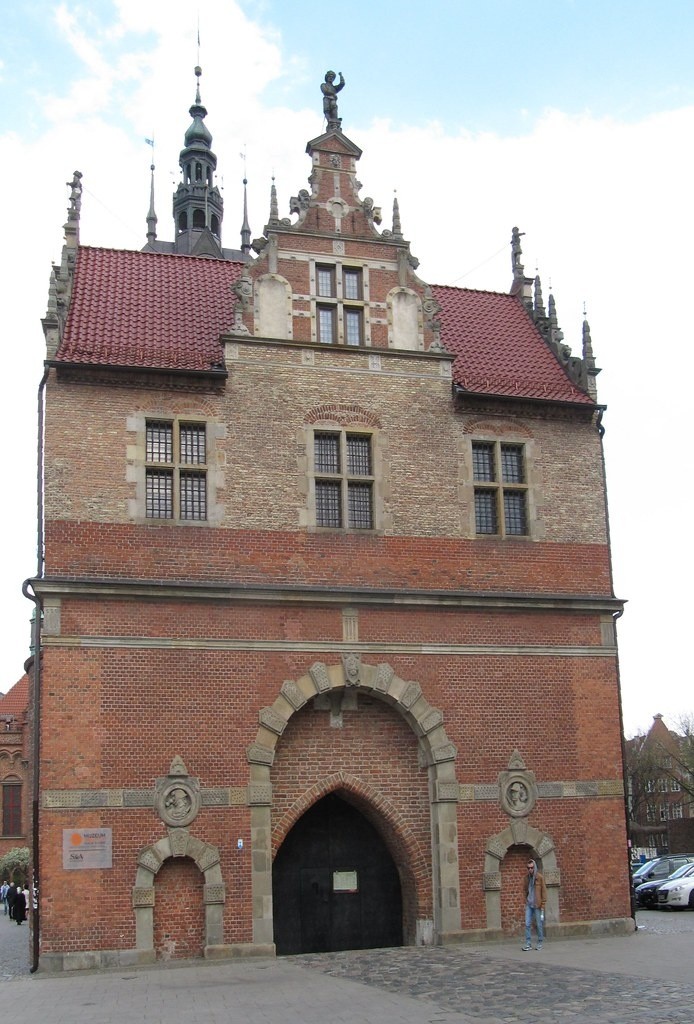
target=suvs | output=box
[632,854,694,888]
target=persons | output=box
[65,170,84,211]
[521,859,547,951]
[509,226,526,267]
[0,880,29,925]
[320,70,345,122]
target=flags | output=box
[145,138,153,146]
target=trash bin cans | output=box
[631,862,644,875]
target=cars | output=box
[634,863,694,909]
[653,864,694,909]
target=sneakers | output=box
[535,944,544,950]
[521,945,533,951]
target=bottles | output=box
[541,911,544,921]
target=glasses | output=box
[527,866,534,870]
